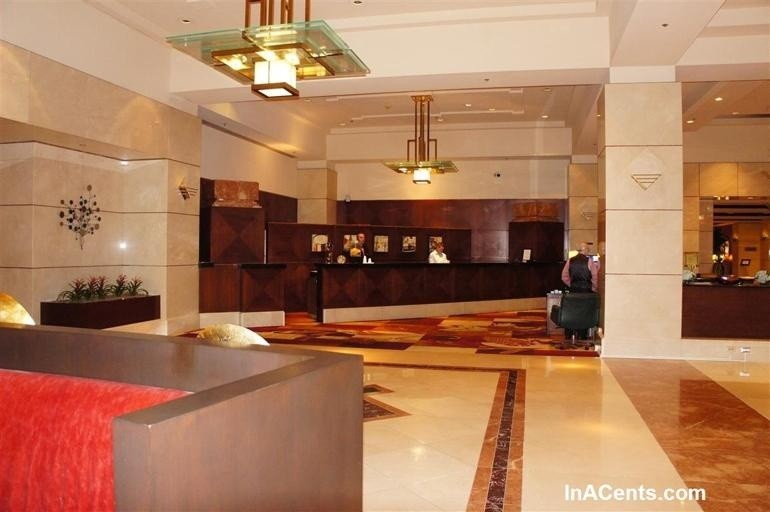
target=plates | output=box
[337,254,346,264]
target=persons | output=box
[561,242,599,340]
[351,233,375,264]
[428,242,451,263]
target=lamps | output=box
[168,1,370,99]
[381,95,458,184]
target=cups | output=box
[550,289,563,295]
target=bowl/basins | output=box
[697,271,754,285]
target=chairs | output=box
[549,290,600,353]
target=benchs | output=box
[3,318,365,510]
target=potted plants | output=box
[40,274,160,329]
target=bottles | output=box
[362,256,366,263]
[367,257,372,262]
[318,242,332,264]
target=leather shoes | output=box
[565,334,588,340]
